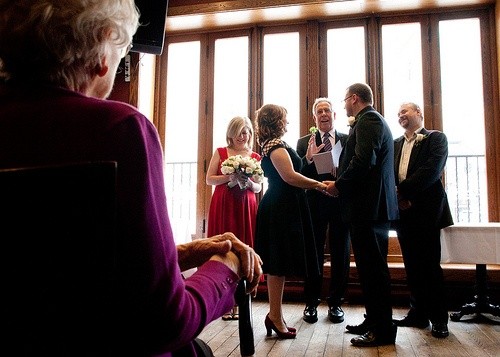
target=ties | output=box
[323,133,332,151]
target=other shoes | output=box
[233,313,239,319]
[222,313,232,320]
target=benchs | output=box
[324,231,500,309]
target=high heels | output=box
[287,325,296,332]
[265,315,296,339]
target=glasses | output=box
[121,39,132,58]
[341,93,357,104]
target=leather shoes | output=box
[303,305,318,323]
[346,319,375,334]
[392,311,429,328]
[432,323,449,337]
[328,304,344,323]
[350,324,397,347]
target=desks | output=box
[441,222,500,322]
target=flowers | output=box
[221,155,264,191]
[346,116,356,127]
[416,133,424,147]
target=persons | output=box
[296,98,349,324]
[205,116,262,320]
[0,0,262,357]
[393,103,454,338]
[322,83,399,347]
[254,104,338,339]
